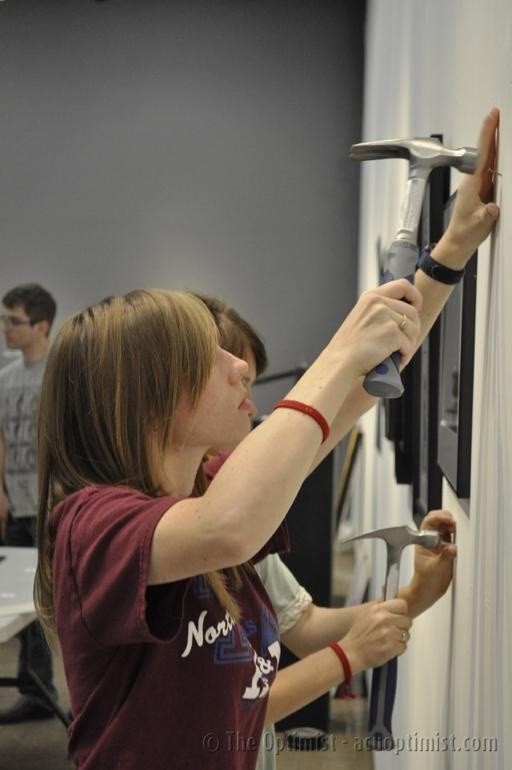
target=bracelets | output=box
[269,396,331,445]
[417,242,466,286]
[328,640,353,685]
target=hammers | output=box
[348,137,479,398]
[341,525,439,752]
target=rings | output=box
[399,314,408,328]
[400,629,406,641]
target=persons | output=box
[33,104,499,770]
[192,288,458,769]
[0,280,64,726]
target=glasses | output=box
[0,315,30,325]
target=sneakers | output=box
[0,698,55,724]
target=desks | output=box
[0,545,73,730]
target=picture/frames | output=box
[384,135,478,533]
